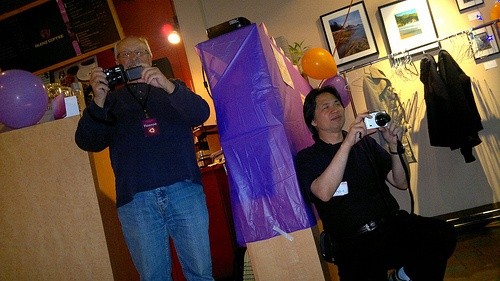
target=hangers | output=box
[343,28,487,92]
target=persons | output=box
[295,87,457,281]
[75,35,215,281]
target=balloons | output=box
[321,76,350,108]
[489,2,500,28]
[0,70,48,129]
[301,47,337,79]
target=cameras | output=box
[104,64,143,87]
[363,110,392,130]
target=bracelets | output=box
[389,147,397,155]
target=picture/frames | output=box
[466,23,500,64]
[320,1,380,69]
[378,0,442,62]
[456,0,484,11]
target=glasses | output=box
[116,50,152,59]
